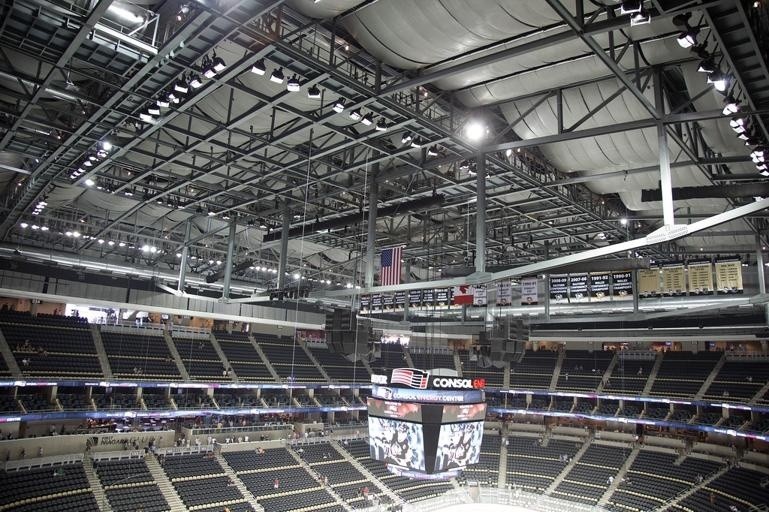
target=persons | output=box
[607,475,615,483]
[1,393,337,460]
[324,476,327,486]
[17,339,49,367]
[224,504,231,512]
[227,367,232,375]
[274,477,279,488]
[357,487,361,497]
[625,476,632,484]
[363,486,369,494]
[51,306,166,325]
[321,473,324,482]
[482,395,751,468]
[223,369,226,375]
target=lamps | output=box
[375,118,391,133]
[620,1,769,182]
[212,56,227,72]
[348,106,364,121]
[287,76,302,93]
[188,73,204,89]
[427,145,439,157]
[167,92,182,106]
[19,138,372,301]
[268,67,286,85]
[400,132,412,144]
[332,96,345,114]
[364,113,375,125]
[201,62,217,80]
[411,136,424,150]
[138,112,155,126]
[459,160,493,181]
[307,83,322,102]
[173,81,190,95]
[156,98,170,110]
[251,58,267,76]
[147,106,162,117]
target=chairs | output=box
[11,311,769,511]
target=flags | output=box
[381,248,401,286]
